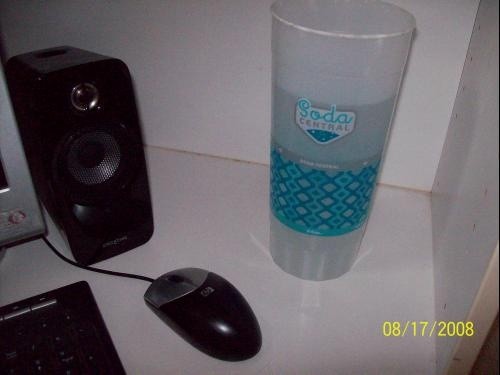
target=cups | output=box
[267,1,419,282]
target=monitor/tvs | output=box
[0,67,47,246]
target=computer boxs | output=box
[7,45,154,263]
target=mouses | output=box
[145,267,263,363]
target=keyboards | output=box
[1,281,127,375]
